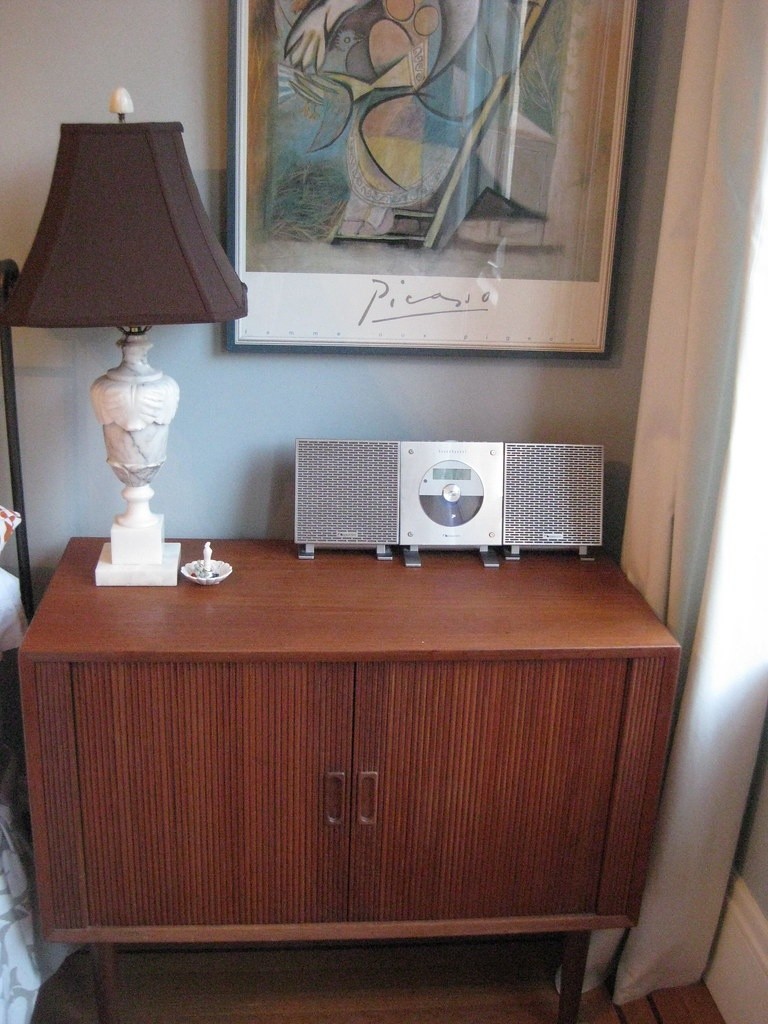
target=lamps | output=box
[6,85,251,587]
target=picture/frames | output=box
[222,0,641,359]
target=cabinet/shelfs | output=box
[17,537,682,1024]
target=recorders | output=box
[293,436,604,561]
